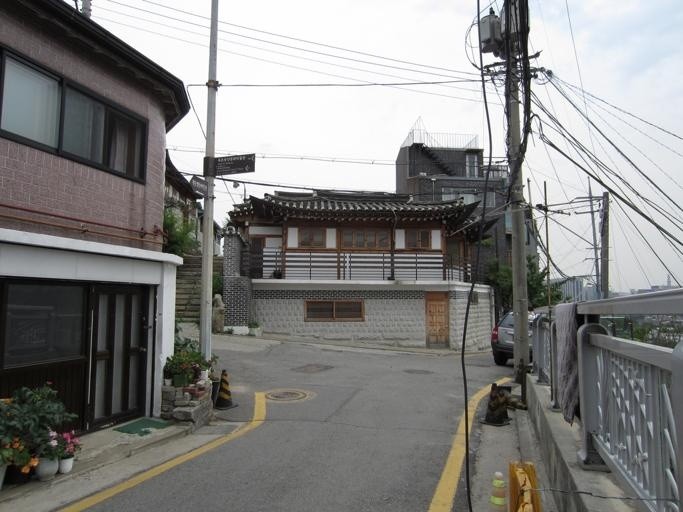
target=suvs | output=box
[492,307,555,368]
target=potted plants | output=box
[164,337,219,409]
[249,322,261,336]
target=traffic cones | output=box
[489,472,508,512]
[217,370,234,410]
[481,382,510,426]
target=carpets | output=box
[112,418,175,436]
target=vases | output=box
[34,457,59,482]
[0,464,8,490]
[9,466,35,484]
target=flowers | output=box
[0,381,83,474]
